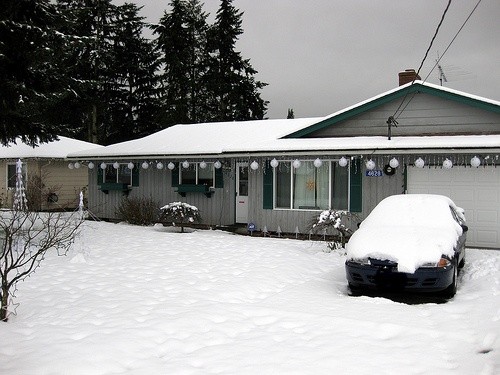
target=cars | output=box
[344,194,468,298]
